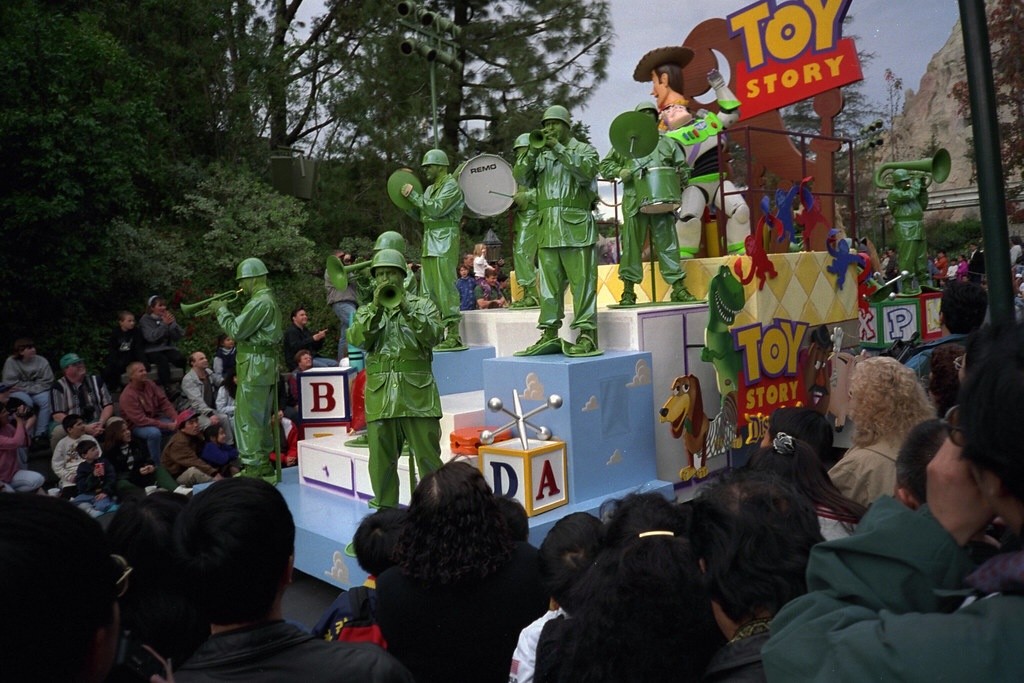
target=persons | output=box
[867,233,1024,302]
[513,106,600,357]
[352,231,418,457]
[180,285,1024,683]
[511,132,543,309]
[347,250,444,512]
[885,171,937,298]
[659,69,752,259]
[208,257,282,482]
[598,101,698,306]
[320,236,508,362]
[632,40,696,127]
[0,291,332,683]
[400,150,465,350]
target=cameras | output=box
[23,408,34,420]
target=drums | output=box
[633,166,682,214]
[453,154,518,219]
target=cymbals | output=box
[609,111,659,160]
[387,170,423,210]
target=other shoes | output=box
[353,434,370,445]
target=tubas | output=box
[875,148,951,190]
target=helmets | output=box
[636,103,659,118]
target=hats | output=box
[176,409,202,427]
[59,353,84,369]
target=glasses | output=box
[941,407,971,448]
[953,356,963,371]
[112,554,133,602]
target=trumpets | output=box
[326,255,373,292]
[181,289,243,318]
[529,129,557,149]
[378,284,402,308]
